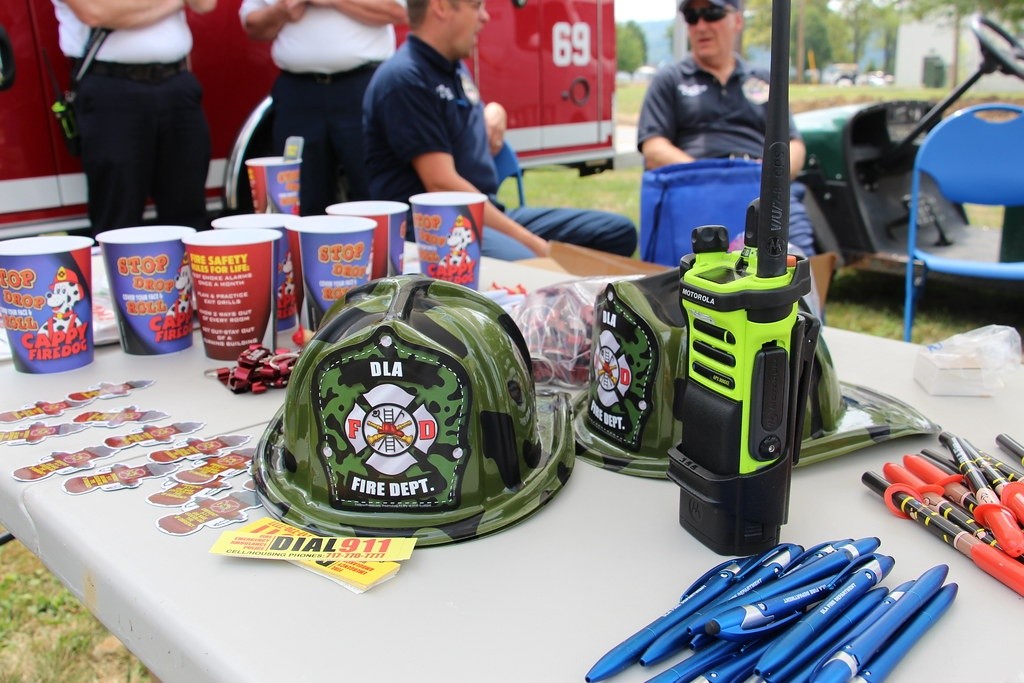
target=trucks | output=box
[2,1,618,238]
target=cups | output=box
[284,215,377,341]
[211,213,302,334]
[409,191,488,292]
[181,229,284,364]
[0,235,95,374]
[325,201,410,281]
[94,225,196,356]
[245,156,303,217]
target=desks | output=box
[0,259,1024,683]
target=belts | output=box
[73,56,188,82]
[280,62,379,83]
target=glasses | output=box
[683,6,736,25]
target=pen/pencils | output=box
[955,436,1024,527]
[882,461,1024,566]
[899,432,1024,536]
[860,471,1024,598]
[949,438,1024,558]
[583,555,758,683]
[638,535,957,683]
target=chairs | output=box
[903,105,1024,343]
[494,143,521,213]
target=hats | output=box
[572,266,941,481]
[253,273,576,549]
[678,0,740,10]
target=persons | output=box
[239,0,411,218]
[362,0,637,262]
[637,0,826,328]
[52,0,218,247]
